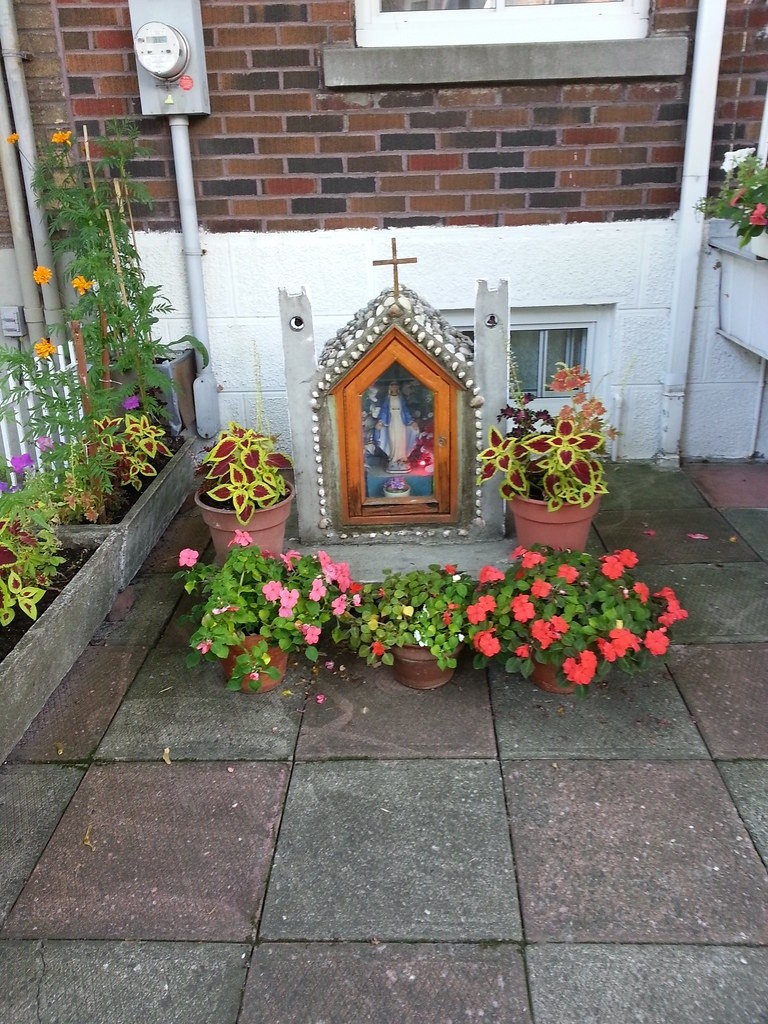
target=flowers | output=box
[0,116,181,521]
[694,146,768,249]
[384,477,408,493]
[469,541,686,688]
[177,531,351,689]
[346,562,480,671]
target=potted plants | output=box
[193,425,297,562]
[0,503,121,768]
[479,418,611,553]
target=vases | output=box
[222,633,290,692]
[30,435,197,592]
[383,486,411,498]
[527,647,580,694]
[390,640,462,691]
